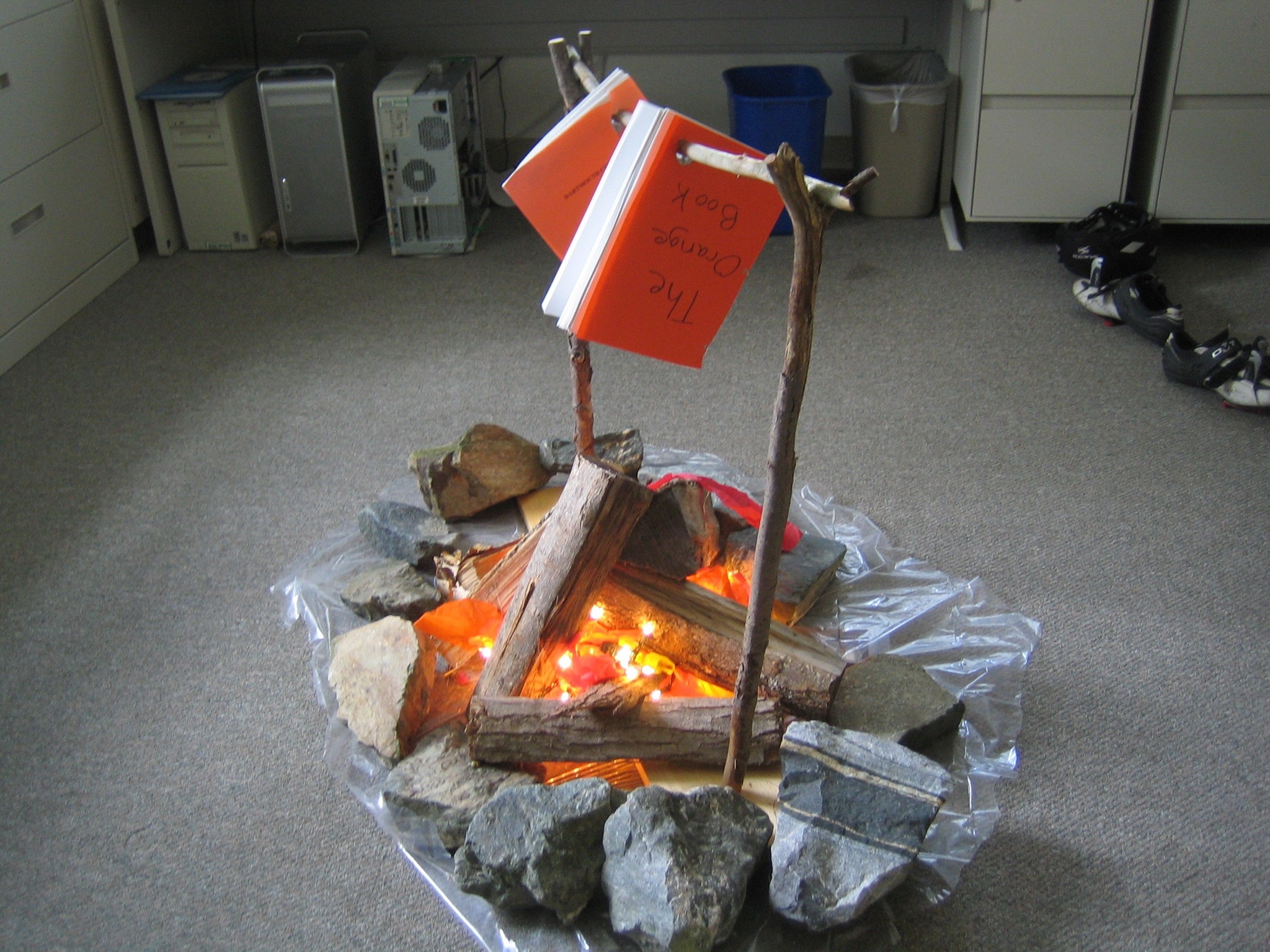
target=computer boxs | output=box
[373,53,488,254]
[154,78,278,252]
[255,29,377,258]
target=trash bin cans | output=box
[843,48,952,219]
[722,64,832,237]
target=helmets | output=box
[1055,202,1166,286]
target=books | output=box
[502,69,648,262]
[540,100,785,369]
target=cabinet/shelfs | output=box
[940,0,1270,251]
[0,0,139,376]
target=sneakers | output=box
[1162,328,1269,412]
[1072,271,1184,345]
